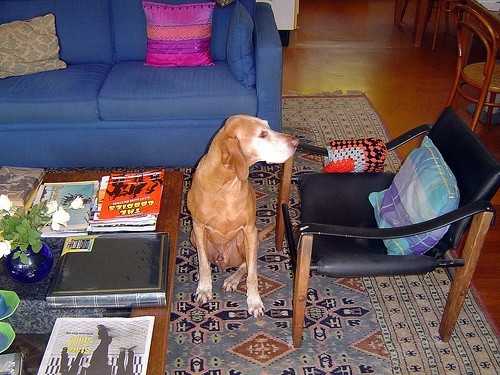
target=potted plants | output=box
[0,204,53,285]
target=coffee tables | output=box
[0,170,187,375]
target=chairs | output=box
[442,4,500,131]
[276,106,500,342]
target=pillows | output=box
[369,135,460,255]
[227,1,255,91]
[141,0,217,67]
[0,13,67,79]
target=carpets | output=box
[45,89,500,375]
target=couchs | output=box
[0,0,283,169]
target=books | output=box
[88,168,164,231]
[33,181,98,237]
[46,232,170,307]
[37,317,155,375]
[0,165,46,215]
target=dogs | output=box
[186,114,300,318]
